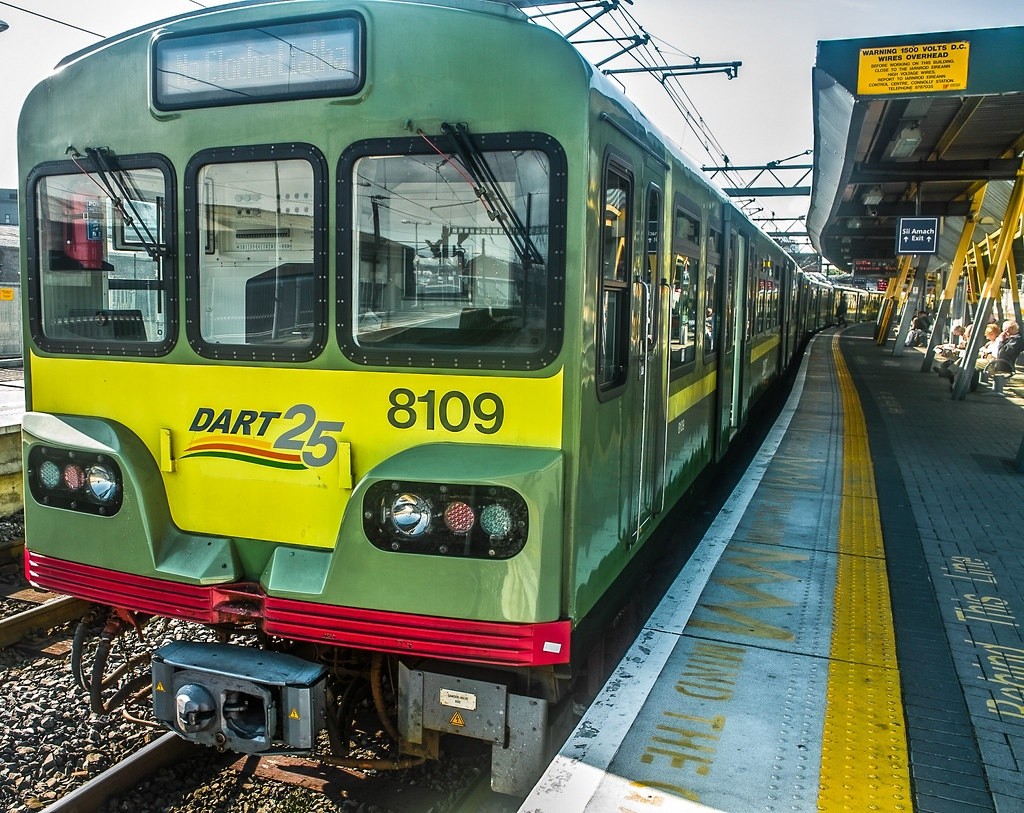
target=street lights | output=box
[401,219,432,307]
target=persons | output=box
[905,311,932,346]
[933,311,1024,391]
[706,308,712,334]
[926,300,934,311]
[838,297,848,328]
[870,297,877,307]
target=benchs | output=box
[976,333,1024,398]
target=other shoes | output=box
[836,325,840,327]
[934,367,948,376]
[844,325,848,328]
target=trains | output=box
[16,0,884,797]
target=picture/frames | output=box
[397,243,475,301]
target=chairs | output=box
[459,308,526,330]
[68,309,147,341]
[245,264,315,346]
